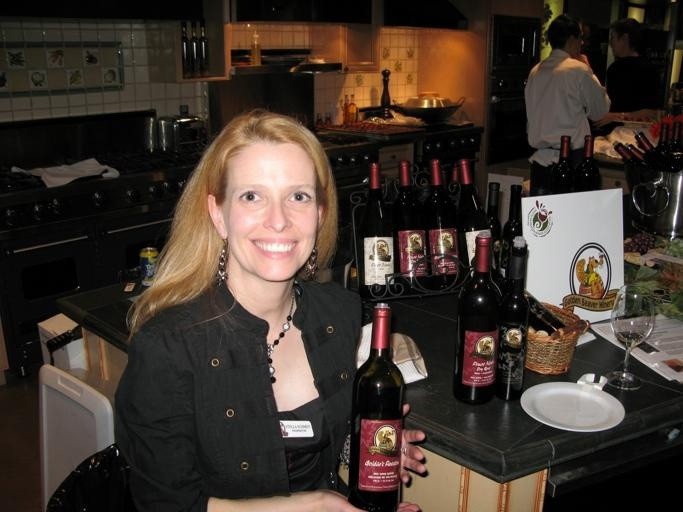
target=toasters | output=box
[170,115,206,151]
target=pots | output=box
[393,92,465,123]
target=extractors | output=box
[230,48,342,78]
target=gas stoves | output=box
[312,130,383,156]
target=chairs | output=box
[40,440,132,512]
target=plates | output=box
[522,382,626,434]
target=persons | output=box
[114,109,429,511]
[523,12,613,196]
[604,18,663,113]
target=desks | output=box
[56,242,683,512]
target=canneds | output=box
[139,247,159,287]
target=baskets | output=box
[526,300,589,376]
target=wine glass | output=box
[604,285,655,392]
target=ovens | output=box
[320,150,380,256]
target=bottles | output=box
[143,116,173,154]
[182,18,209,78]
[453,230,529,402]
[347,304,404,512]
[380,70,390,119]
[523,289,566,338]
[555,123,683,193]
[316,94,358,128]
[359,161,527,297]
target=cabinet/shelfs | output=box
[305,23,380,76]
[378,141,412,187]
[143,0,230,87]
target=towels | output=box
[355,319,429,386]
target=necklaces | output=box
[267,279,300,383]
